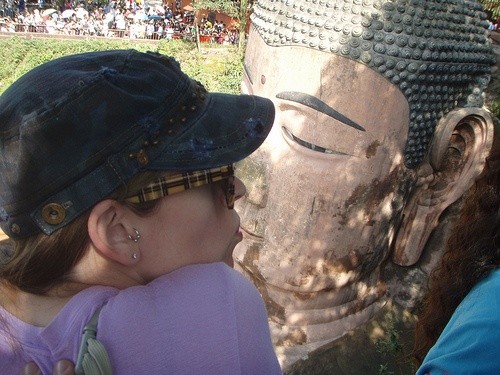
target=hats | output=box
[0,50,275,244]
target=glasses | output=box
[125,154,237,209]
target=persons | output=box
[21,267,499,374]
[484,16,499,31]
[232,0,497,325]
[0,0,242,47]
[0,47,286,375]
